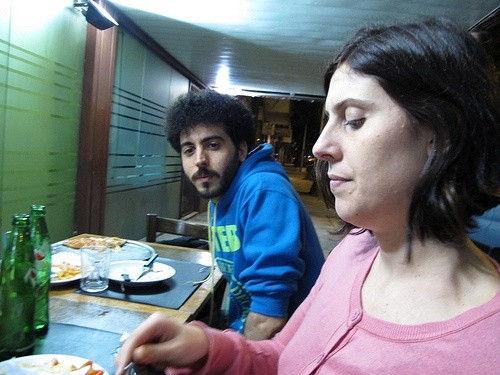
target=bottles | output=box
[0,214,36,361]
[30,205,51,338]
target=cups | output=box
[80,246,112,292]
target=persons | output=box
[115,19,500,375]
[164,90,325,340]
[274,134,301,166]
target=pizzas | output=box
[63,235,127,249]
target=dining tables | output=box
[0,232,232,375]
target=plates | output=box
[79,238,154,261]
[0,354,110,375]
[50,252,94,287]
[100,260,176,287]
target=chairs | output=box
[143,213,218,253]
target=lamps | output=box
[74,0,121,34]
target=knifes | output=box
[136,253,158,280]
